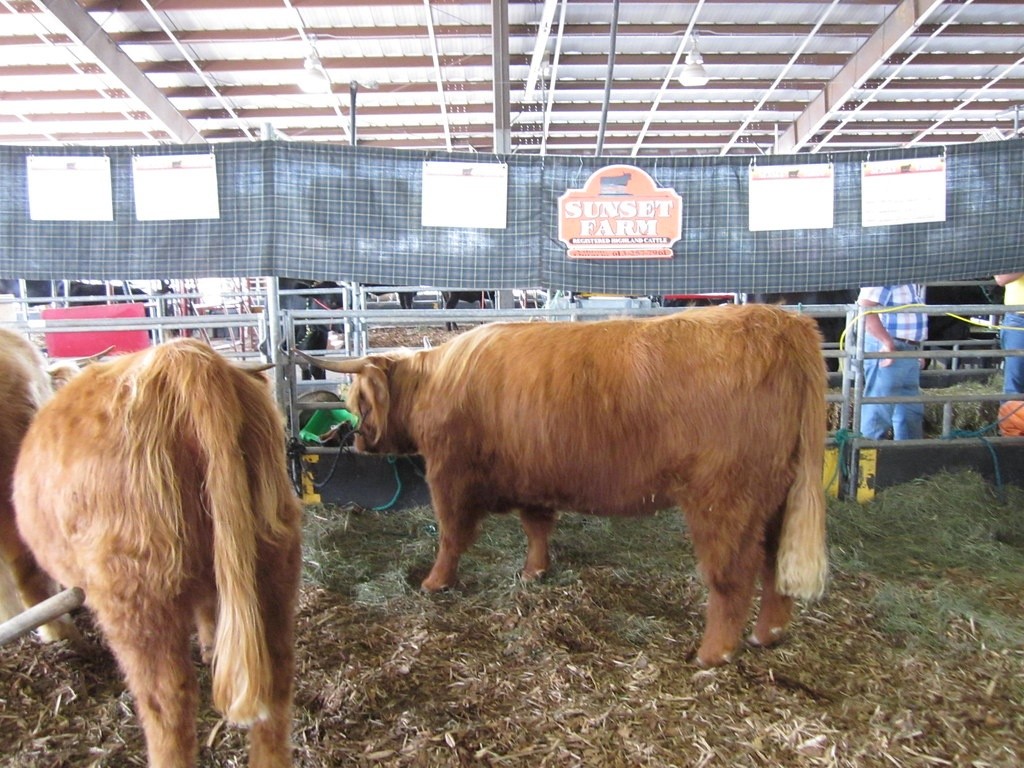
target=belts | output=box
[892,336,921,347]
[1009,310,1024,318]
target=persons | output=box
[853,283,928,443]
[991,273,1023,403]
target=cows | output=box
[288,302,832,675]
[0,325,304,768]
